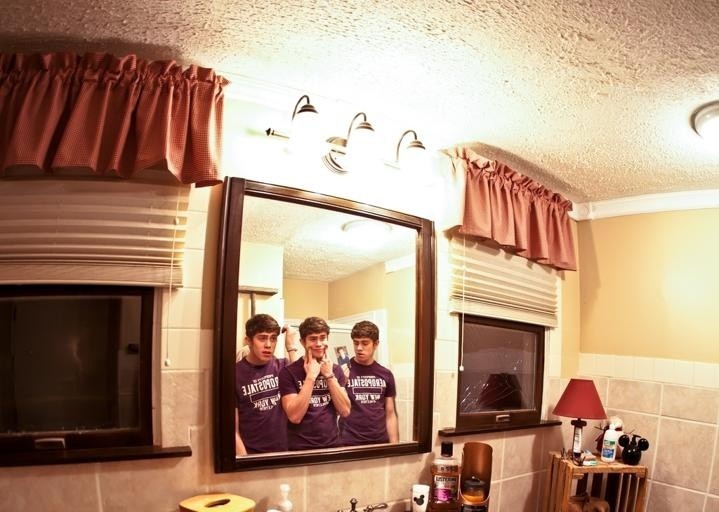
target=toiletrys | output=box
[278,484,294,512]
[618,434,649,465]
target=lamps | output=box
[553,375,607,461]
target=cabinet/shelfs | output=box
[540,448,649,511]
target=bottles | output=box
[428,440,462,504]
[600,422,620,463]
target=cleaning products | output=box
[432,441,460,512]
[600,420,617,462]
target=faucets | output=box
[349,499,388,512]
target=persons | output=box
[277,316,351,450]
[337,320,399,445]
[236,313,300,455]
[337,347,349,365]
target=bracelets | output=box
[287,346,299,352]
[326,372,335,380]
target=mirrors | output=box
[212,174,436,472]
